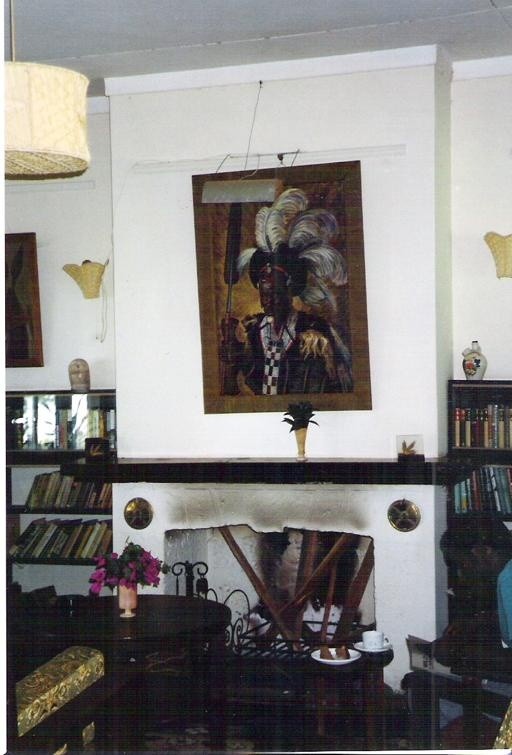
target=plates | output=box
[353,641,391,652]
[311,646,359,666]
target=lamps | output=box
[63,259,110,298]
[484,233,512,279]
[4,1,92,180]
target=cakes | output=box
[320,645,335,661]
[337,646,350,660]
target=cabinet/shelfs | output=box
[6,390,116,622]
[449,379,512,527]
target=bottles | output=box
[463,341,487,380]
[67,359,91,392]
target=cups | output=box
[362,631,390,648]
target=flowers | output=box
[89,543,163,595]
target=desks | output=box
[301,642,394,749]
[35,595,231,753]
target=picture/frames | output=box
[5,232,43,367]
[191,161,372,414]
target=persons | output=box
[421,516,511,734]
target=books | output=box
[449,403,511,516]
[7,470,114,559]
[403,636,463,684]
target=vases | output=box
[119,583,138,618]
